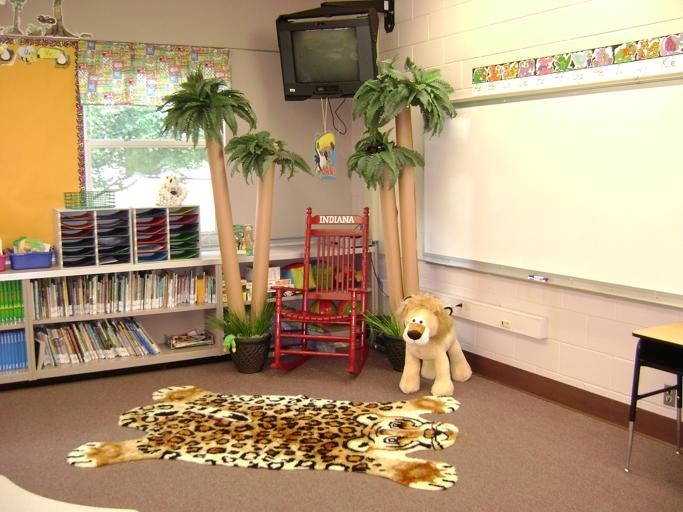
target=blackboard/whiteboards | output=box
[416,72,683,309]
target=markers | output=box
[529,275,548,281]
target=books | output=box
[0,279,27,375]
[31,268,216,374]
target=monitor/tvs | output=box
[274,5,380,103]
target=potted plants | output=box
[343,49,457,369]
[153,62,321,376]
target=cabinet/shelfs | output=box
[53,202,201,270]
[0,242,380,387]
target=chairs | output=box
[268,204,371,382]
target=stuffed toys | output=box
[392,293,473,398]
[153,173,188,209]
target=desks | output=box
[623,324,682,473]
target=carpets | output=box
[63,383,461,493]
